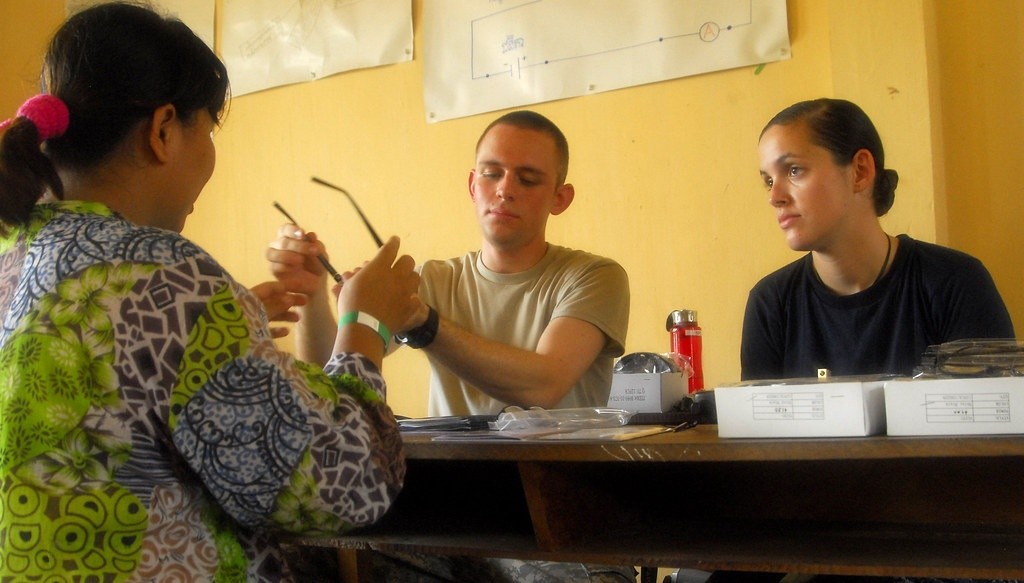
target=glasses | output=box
[494,406,547,421]
[612,351,680,374]
[919,340,1024,378]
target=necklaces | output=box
[808,234,892,296]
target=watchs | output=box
[393,304,438,350]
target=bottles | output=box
[665,309,704,396]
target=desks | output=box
[286,427,1024,583]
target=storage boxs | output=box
[884,377,1024,434]
[607,371,691,414]
[715,379,887,439]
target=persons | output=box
[706,96,1024,582]
[0,3,420,582]
[263,110,642,583]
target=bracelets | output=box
[341,310,390,356]
